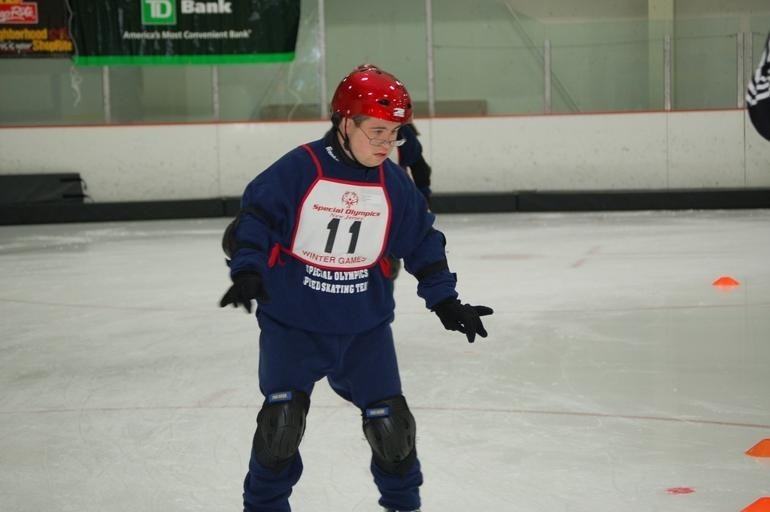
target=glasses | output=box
[353,120,408,147]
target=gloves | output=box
[435,298,494,343]
[220,273,271,313]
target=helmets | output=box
[331,64,413,123]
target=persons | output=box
[216,69,496,511]
[356,63,433,286]
